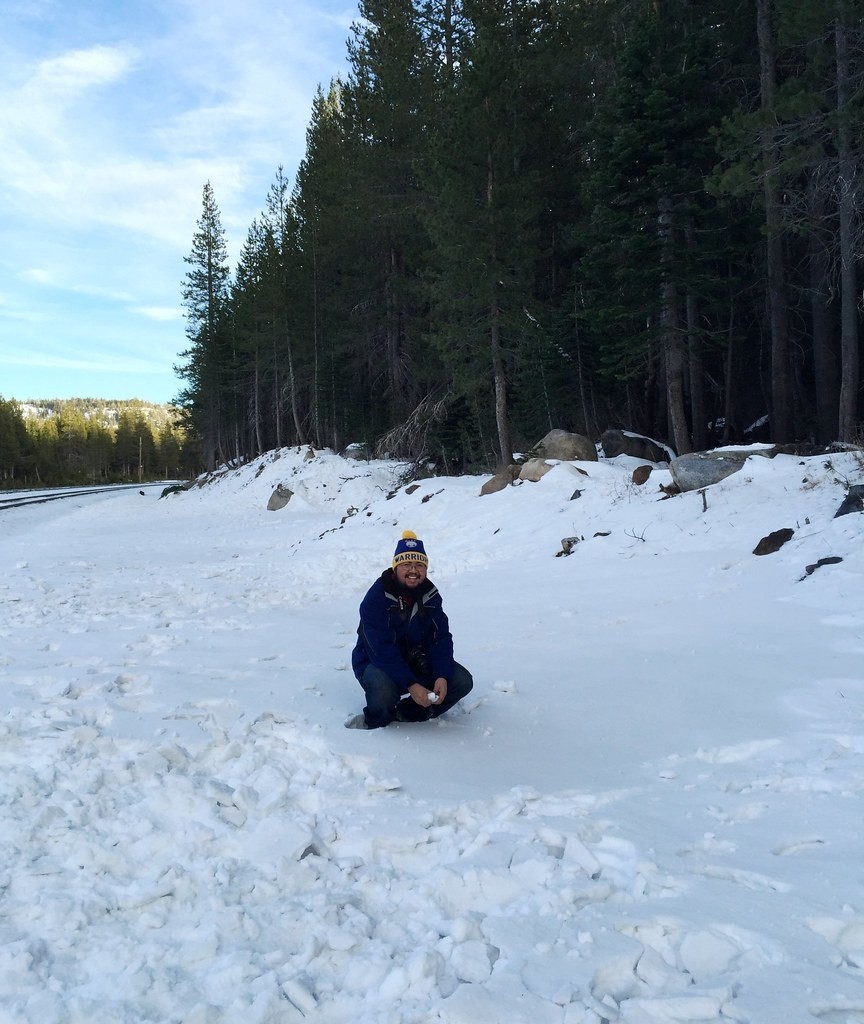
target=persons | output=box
[350,530,474,729]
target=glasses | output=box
[398,563,425,569]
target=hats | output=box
[392,530,428,570]
